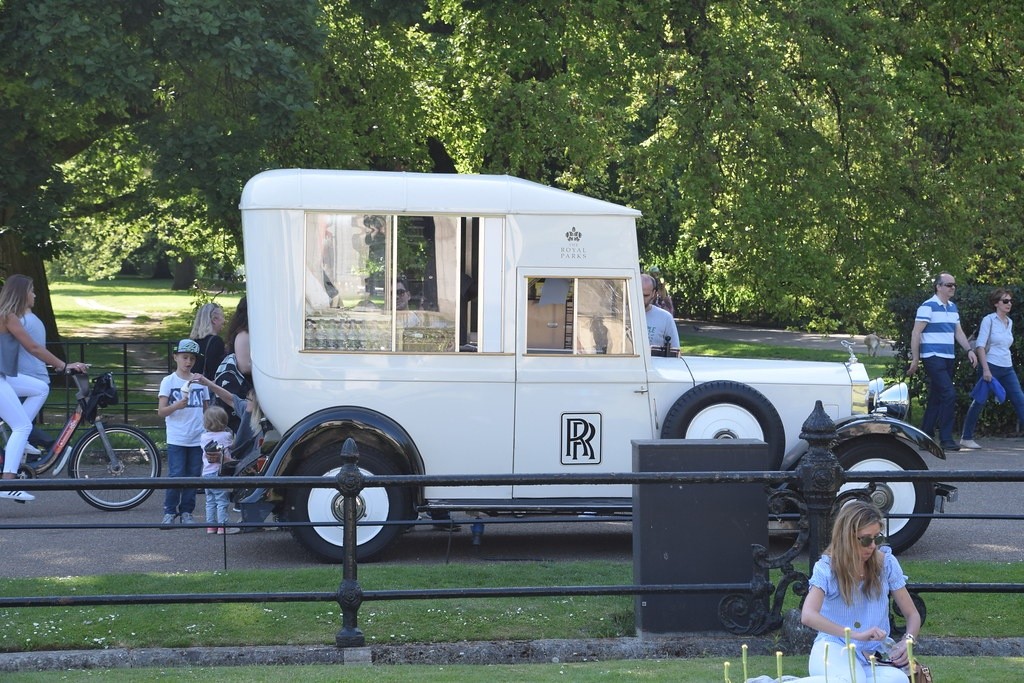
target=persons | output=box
[383,278,419,328]
[640,266,680,351]
[0,273,89,500]
[188,373,264,513]
[960,288,1024,449]
[352,300,380,338]
[908,271,977,451]
[785,501,921,683]
[200,406,240,534]
[157,339,210,528]
[188,294,254,431]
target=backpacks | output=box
[214,353,248,423]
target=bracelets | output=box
[64,363,67,370]
[904,633,916,644]
[967,349,972,356]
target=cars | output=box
[217,171,959,560]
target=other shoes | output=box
[960,438,982,449]
[232,503,241,511]
[919,446,925,450]
[940,442,961,452]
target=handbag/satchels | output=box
[904,659,933,683]
[85,370,119,423]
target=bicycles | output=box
[0,362,162,515]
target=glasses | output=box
[998,299,1014,304]
[210,303,219,314]
[857,533,885,547]
[390,289,408,296]
[938,282,958,288]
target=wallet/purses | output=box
[204,440,221,463]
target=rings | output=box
[77,365,79,367]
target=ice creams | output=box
[181,381,190,399]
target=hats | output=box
[174,339,204,357]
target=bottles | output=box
[881,637,916,676]
[305,319,455,353]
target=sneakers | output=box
[217,527,241,534]
[4,441,41,454]
[179,511,195,524]
[0,490,35,500]
[207,527,217,534]
[160,513,177,530]
